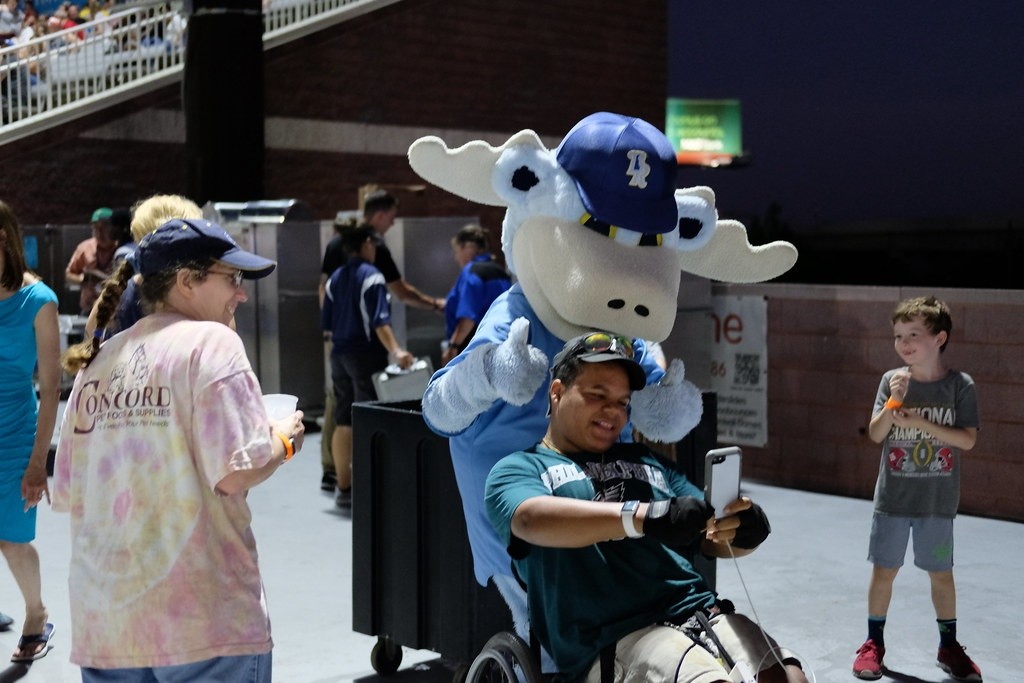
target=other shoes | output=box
[335,488,352,509]
[320,471,336,488]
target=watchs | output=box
[621,500,644,538]
[281,432,296,464]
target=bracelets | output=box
[433,297,437,310]
[885,395,903,412]
[388,348,401,361]
[447,343,463,349]
[276,431,294,460]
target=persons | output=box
[485,331,811,683]
[320,183,445,510]
[50,218,305,683]
[0,0,187,119]
[65,195,235,349]
[441,224,513,364]
[852,298,985,683]
[0,200,60,660]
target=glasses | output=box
[198,268,243,288]
[556,333,633,380]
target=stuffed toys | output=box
[408,111,799,683]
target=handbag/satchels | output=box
[372,356,435,402]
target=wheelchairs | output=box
[466,559,547,683]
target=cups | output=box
[261,393,299,421]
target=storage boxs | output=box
[352,397,515,662]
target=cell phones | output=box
[705,446,742,520]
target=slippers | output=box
[11,622,55,660]
[0,612,14,629]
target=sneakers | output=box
[936,642,982,681]
[851,638,885,678]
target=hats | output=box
[334,210,356,225]
[90,208,113,224]
[551,332,646,391]
[134,218,277,281]
